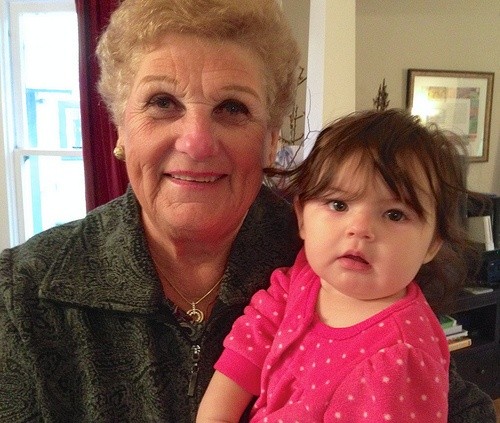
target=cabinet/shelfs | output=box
[427,191,500,398]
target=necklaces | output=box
[152,256,222,321]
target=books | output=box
[441,314,472,351]
[463,286,493,295]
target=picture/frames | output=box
[406,69,495,163]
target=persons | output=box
[0,0,496,423]
[195,108,474,423]
